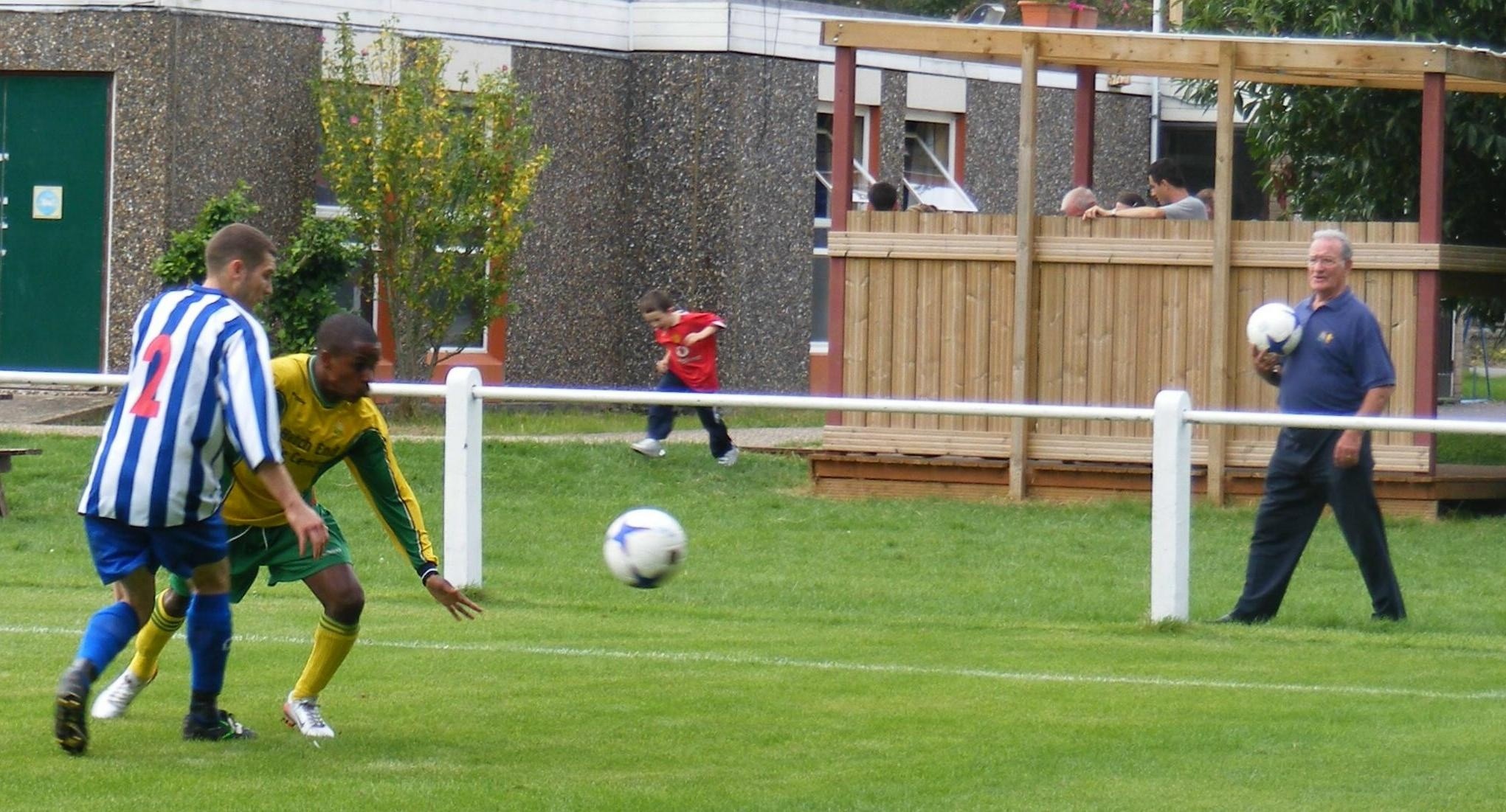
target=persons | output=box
[1060,158,1215,221]
[1215,229,1406,625]
[90,314,482,737]
[628,291,739,467]
[866,181,899,212]
[51,223,330,756]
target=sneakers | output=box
[718,447,740,466]
[91,662,158,718]
[182,710,259,741]
[631,439,662,457]
[282,692,335,737]
[55,696,87,754]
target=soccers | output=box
[1247,301,1304,359]
[603,507,686,589]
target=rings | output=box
[1346,455,1351,461]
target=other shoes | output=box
[1216,609,1250,623]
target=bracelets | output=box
[1112,208,1118,216]
